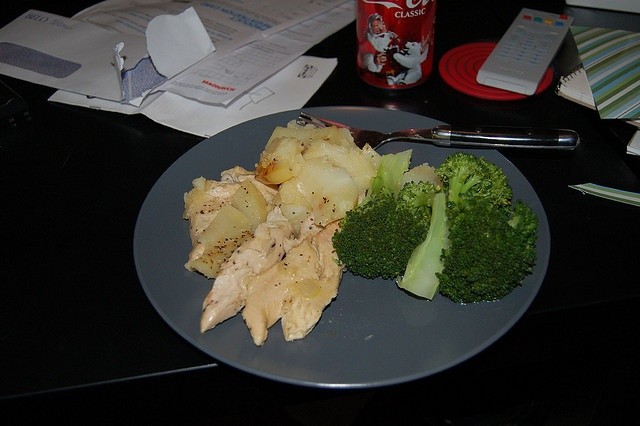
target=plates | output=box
[132,103,550,388]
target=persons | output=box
[356,12,402,78]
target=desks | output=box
[1,0,640,426]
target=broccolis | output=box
[331,147,537,302]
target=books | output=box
[556,61,640,128]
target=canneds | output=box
[356,0,436,91]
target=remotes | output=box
[477,8,574,96]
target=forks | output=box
[298,110,581,151]
